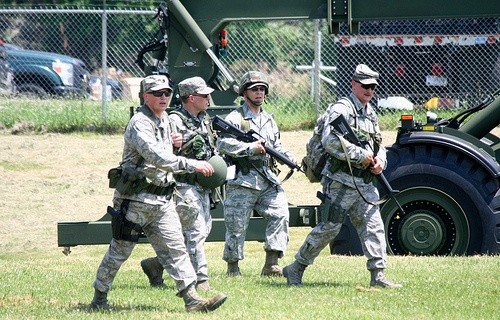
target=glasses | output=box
[355,79,375,90]
[244,85,266,91]
[147,90,171,97]
[193,93,208,98]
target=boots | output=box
[281,261,307,286]
[261,249,282,276]
[180,284,227,313]
[370,268,403,289]
[227,261,241,276]
[141,256,168,289]
[89,289,110,313]
[197,282,210,293]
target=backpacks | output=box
[301,105,332,183]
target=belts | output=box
[246,158,274,168]
[145,184,175,201]
[172,172,198,184]
[330,156,375,184]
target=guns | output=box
[106,206,122,239]
[329,114,407,215]
[211,114,308,178]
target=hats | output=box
[178,76,214,97]
[352,64,379,86]
[143,75,173,93]
[239,71,269,96]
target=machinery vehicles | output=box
[57,0,500,259]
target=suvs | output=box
[0,41,90,99]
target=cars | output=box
[85,74,124,99]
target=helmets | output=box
[198,155,228,188]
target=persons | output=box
[282,64,403,289]
[140,76,221,292]
[217,71,297,276]
[88,75,227,312]
